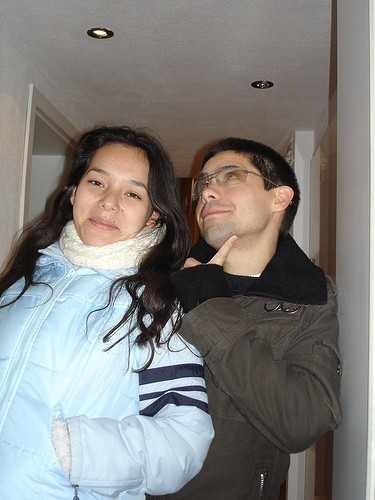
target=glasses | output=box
[192,167,279,201]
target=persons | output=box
[145,136,340,499]
[2,124,215,500]
